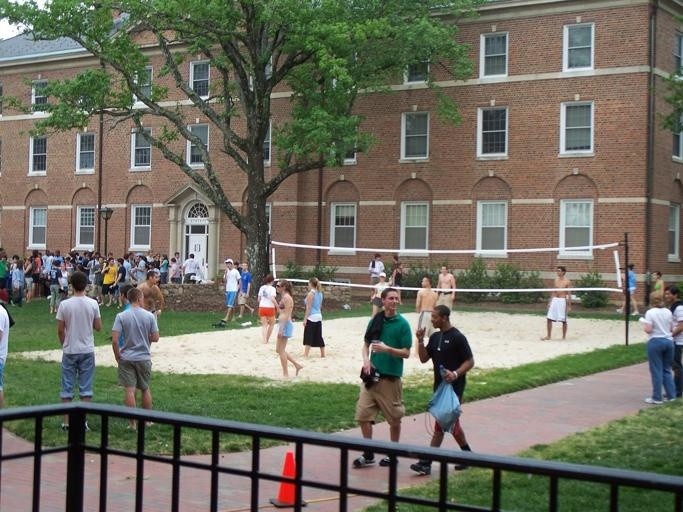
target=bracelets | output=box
[452,370,459,378]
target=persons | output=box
[352,288,412,466]
[112,288,160,431]
[117,285,133,348]
[411,305,474,475]
[220,254,456,358]
[639,271,682,404]
[541,266,571,340]
[56,272,102,430]
[271,280,303,378]
[616,264,639,316]
[0,306,9,412]
[0,248,199,318]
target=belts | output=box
[380,374,400,382]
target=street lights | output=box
[97,207,113,260]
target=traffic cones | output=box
[269,451,306,509]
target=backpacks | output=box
[425,380,461,438]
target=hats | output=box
[224,258,233,264]
[380,272,387,278]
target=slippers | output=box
[380,456,398,466]
[353,456,375,467]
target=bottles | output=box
[440,365,448,383]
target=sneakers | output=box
[127,421,154,431]
[644,392,682,404]
[411,463,430,474]
[61,421,90,433]
[617,309,640,316]
[455,464,468,470]
